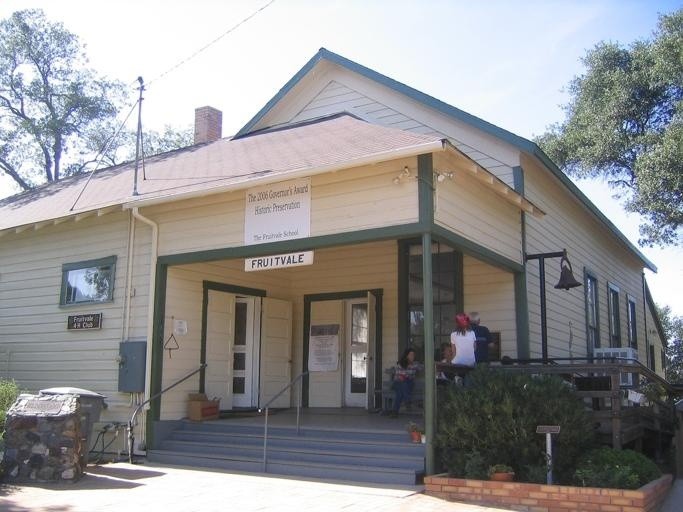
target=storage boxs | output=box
[185,393,221,421]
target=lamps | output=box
[437,168,456,181]
[391,164,411,185]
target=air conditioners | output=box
[592,347,639,387]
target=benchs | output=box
[374,368,425,415]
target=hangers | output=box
[163,319,179,350]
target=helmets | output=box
[456,313,470,328]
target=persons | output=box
[436,311,492,386]
[379,349,424,416]
